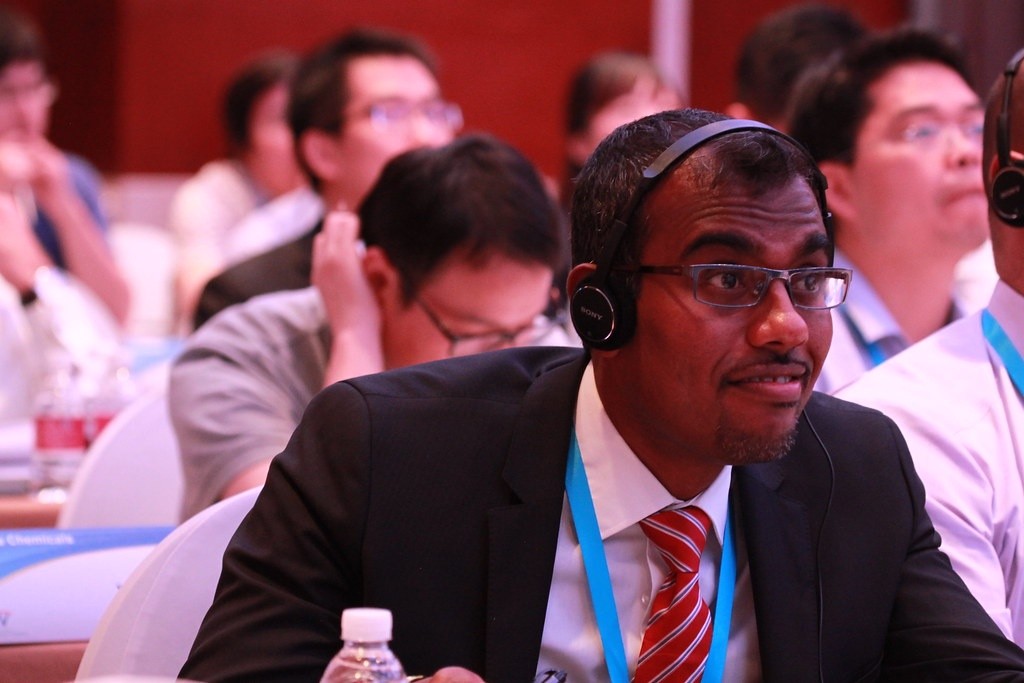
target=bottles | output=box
[318,607,409,683]
[32,349,130,503]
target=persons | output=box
[194,30,462,336]
[177,109,1024,683]
[828,48,1024,650]
[787,26,989,396]
[168,134,567,526]
[0,14,128,385]
[559,54,675,201]
[728,7,873,138]
[168,60,325,338]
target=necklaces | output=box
[839,304,886,365]
[563,432,736,682]
[982,308,1024,402]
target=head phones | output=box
[986,49,1024,228]
[571,119,843,351]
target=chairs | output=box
[0,174,268,683]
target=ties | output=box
[630,505,714,683]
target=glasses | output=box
[614,263,853,309]
[403,279,555,358]
[341,101,464,137]
